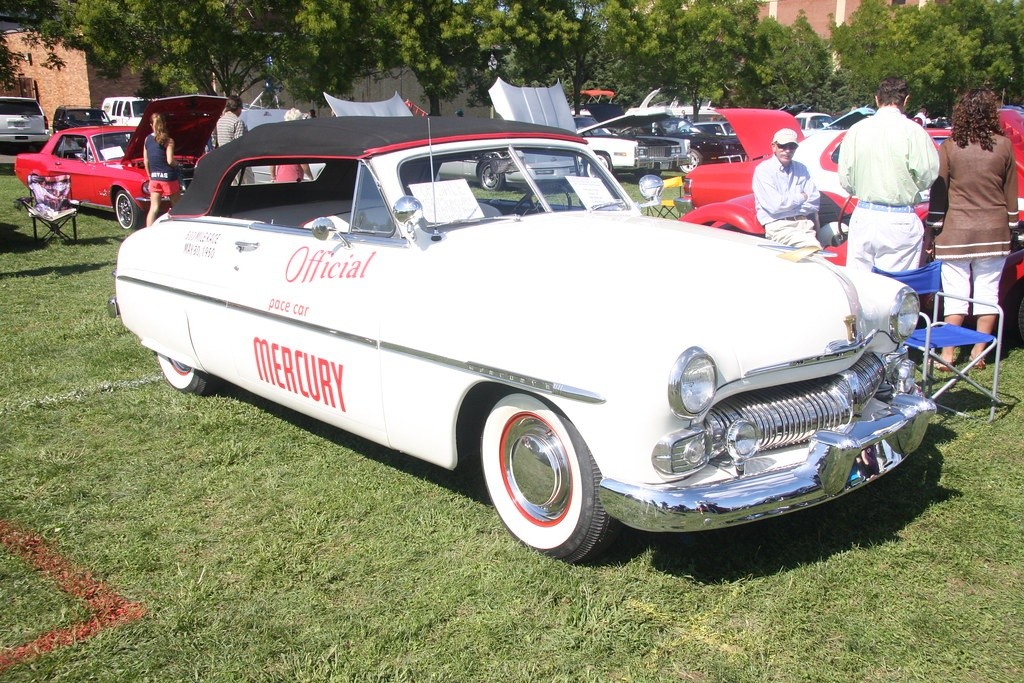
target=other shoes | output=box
[964,354,986,369]
[927,358,953,372]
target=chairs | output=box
[13,170,87,246]
[867,259,1015,425]
[643,173,685,219]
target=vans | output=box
[101,96,159,126]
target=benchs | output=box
[232,178,385,227]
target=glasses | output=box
[774,142,797,149]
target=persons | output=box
[217,95,255,186]
[679,110,692,123]
[752,128,824,251]
[838,77,939,272]
[271,108,313,182]
[914,108,927,128]
[143,112,182,227]
[924,87,1020,370]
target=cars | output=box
[0,97,50,144]
[107,117,936,564]
[673,106,1024,346]
[322,78,754,192]
[53,105,117,136]
[14,94,229,231]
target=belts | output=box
[779,215,808,221]
[856,200,915,213]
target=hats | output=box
[772,128,799,148]
